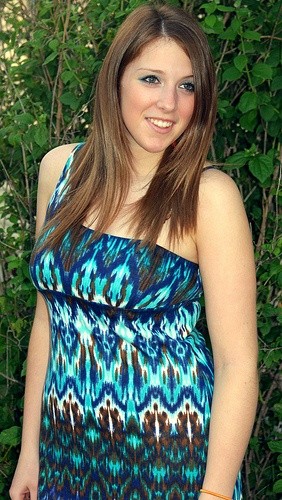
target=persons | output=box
[7,2,259,499]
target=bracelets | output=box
[199,486,233,499]
